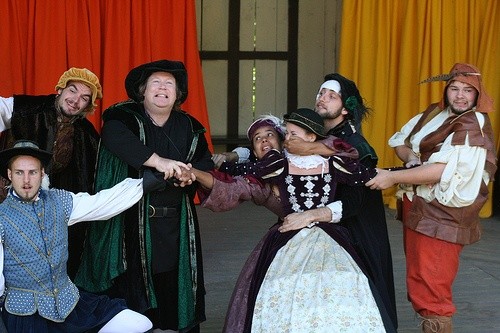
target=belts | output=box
[148,205,180,218]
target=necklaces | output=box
[146,112,160,127]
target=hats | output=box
[246,113,286,141]
[417,63,495,112]
[55,67,103,106]
[0,139,53,180]
[324,73,375,125]
[283,108,329,139]
[125,59,188,105]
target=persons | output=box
[0,68,104,294]
[0,140,166,332]
[71,57,215,331]
[171,114,359,332]
[211,72,401,332]
[359,62,499,332]
[216,106,396,332]
